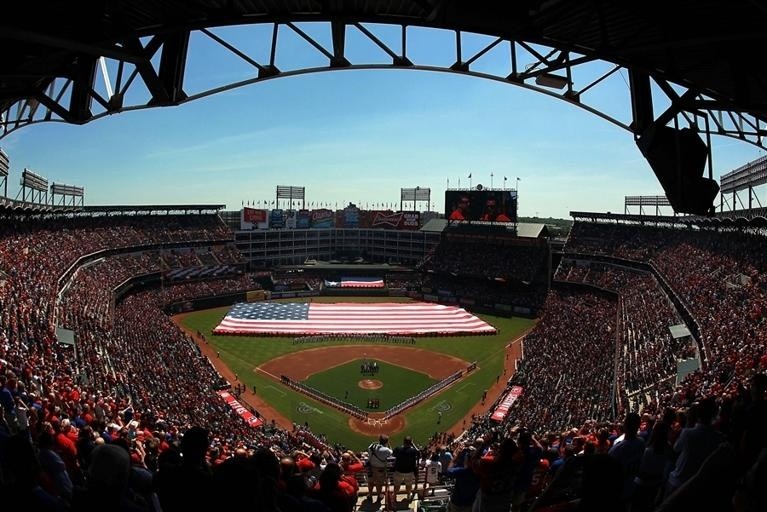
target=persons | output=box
[0,209,365,512]
[366,213,765,511]
[448,195,512,222]
